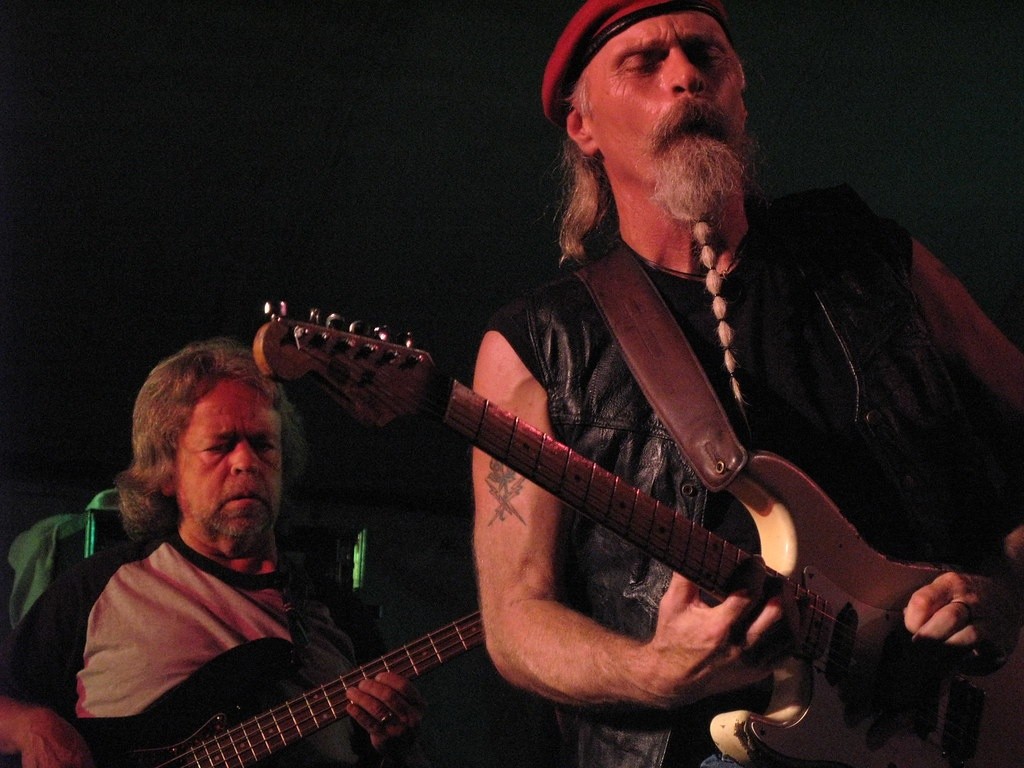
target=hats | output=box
[542,0,727,129]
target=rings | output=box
[950,599,973,622]
[377,712,393,726]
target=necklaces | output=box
[641,256,736,278]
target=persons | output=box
[1,339,428,768]
[472,1,1024,768]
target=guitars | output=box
[252,300,1024,768]
[1,603,485,768]
